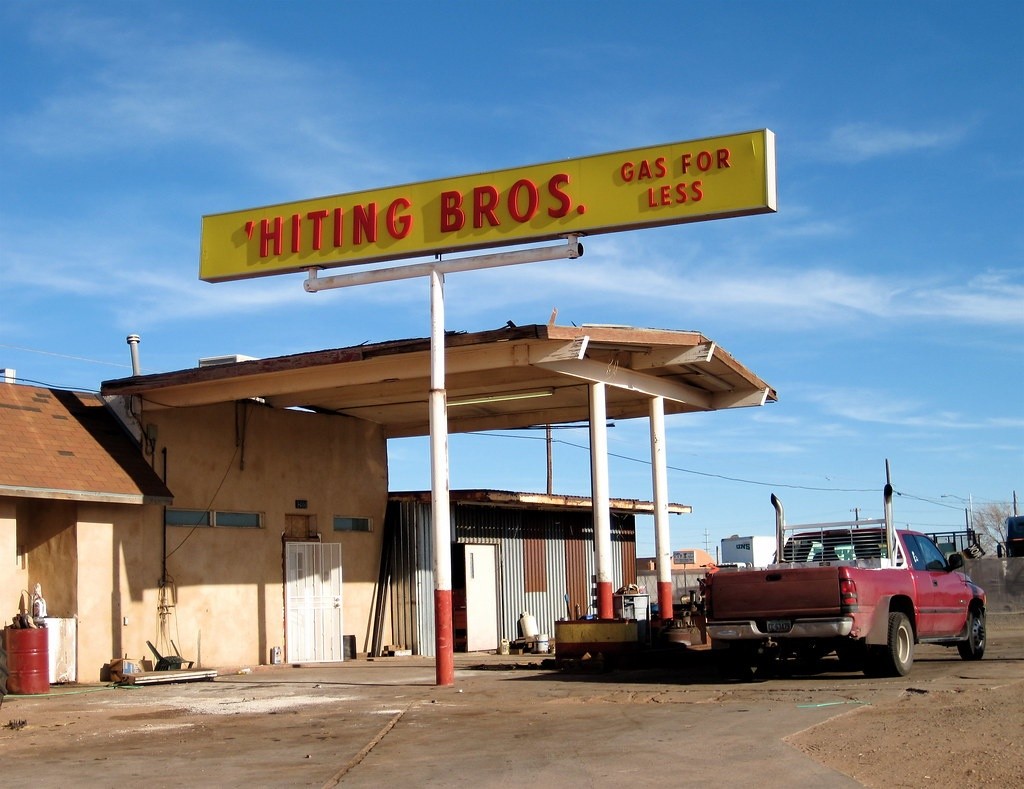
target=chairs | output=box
[813,551,841,561]
[145,639,194,671]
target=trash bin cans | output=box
[8,628,49,695]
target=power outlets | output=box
[124,617,129,625]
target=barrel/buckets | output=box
[500,639,510,655]
[536,634,548,652]
[5,627,49,696]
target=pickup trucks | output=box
[704,484,987,676]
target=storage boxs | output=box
[110,659,153,683]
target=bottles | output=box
[520,612,539,639]
